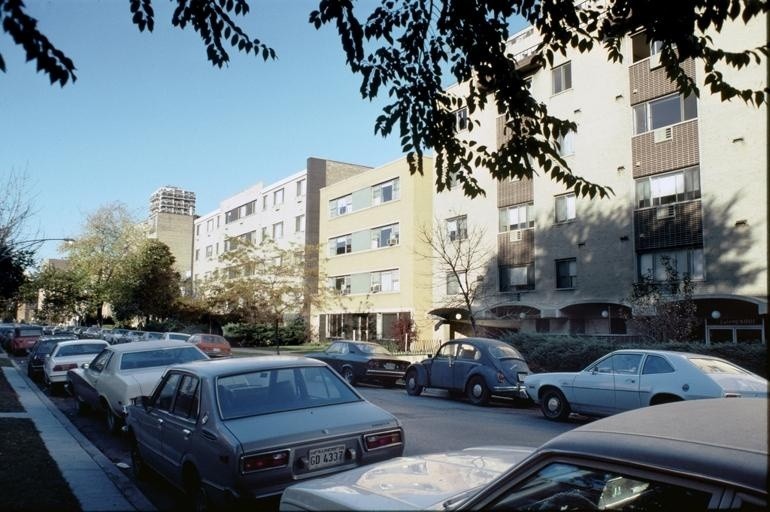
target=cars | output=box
[523,347,746,420]
[406,334,538,408]
[276,396,747,511]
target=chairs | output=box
[461,349,472,358]
[269,380,296,403]
